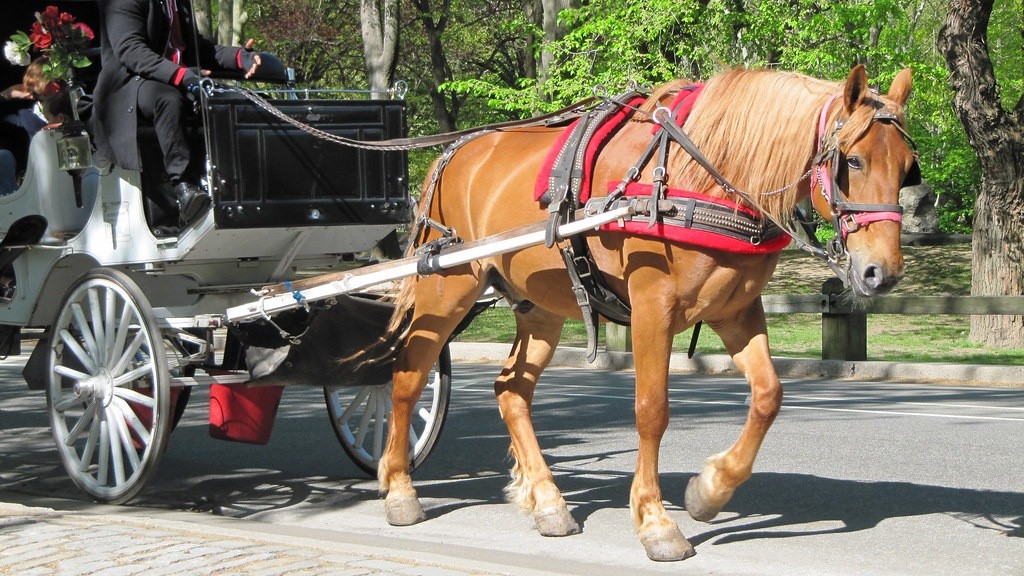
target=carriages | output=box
[0,65,919,561]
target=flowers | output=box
[0,5,100,102]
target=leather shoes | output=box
[176,181,208,221]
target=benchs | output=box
[85,45,293,233]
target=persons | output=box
[92,0,260,223]
[0,56,72,194]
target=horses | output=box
[376,63,915,562]
